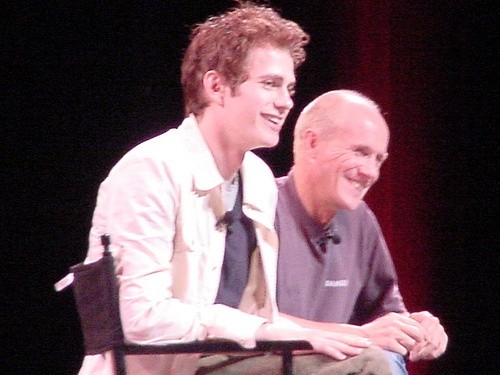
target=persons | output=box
[272,88,448,375]
[77,0,398,374]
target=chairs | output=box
[71,234,313,375]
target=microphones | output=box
[222,212,234,225]
[333,236,340,244]
